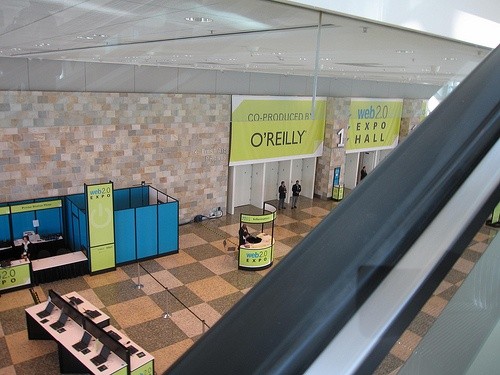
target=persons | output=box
[360,166,367,180]
[20,235,35,260]
[291,180,301,209]
[238,224,250,251]
[277,181,287,210]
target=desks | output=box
[25,291,155,375]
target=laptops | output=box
[90,344,111,366]
[127,345,138,356]
[108,330,122,340]
[83,308,102,318]
[50,313,68,330]
[72,331,92,352]
[67,296,83,305]
[36,301,55,319]
